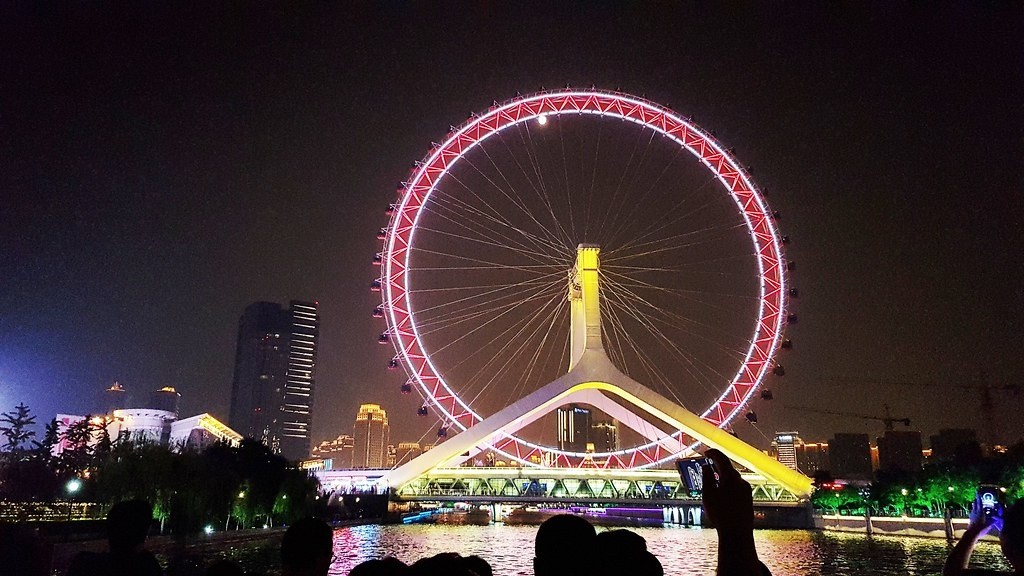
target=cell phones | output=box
[675,456,720,497]
[979,484,999,522]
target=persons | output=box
[0,449,774,576]
[943,497,1024,576]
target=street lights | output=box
[836,493,840,514]
[66,480,81,522]
[901,488,909,516]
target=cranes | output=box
[785,402,912,431]
